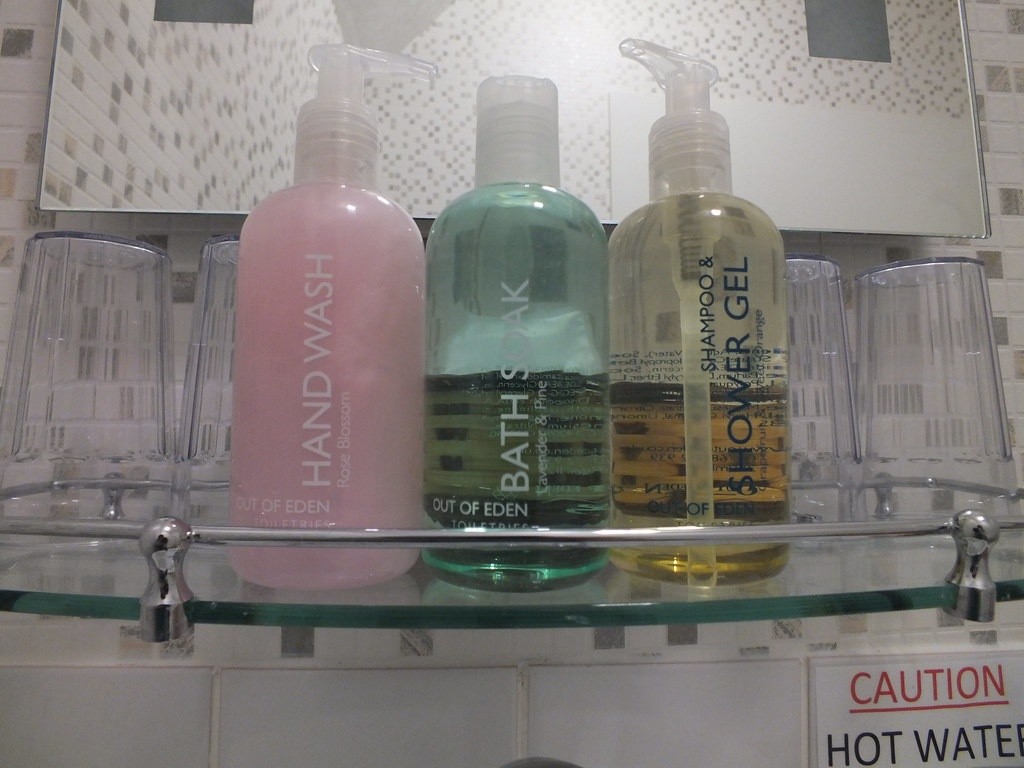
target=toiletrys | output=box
[228,43,421,588]
[419,76,615,592]
[606,37,793,583]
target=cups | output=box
[853,257,1024,547]
[0,231,180,550]
[180,232,241,528]
[783,252,865,547]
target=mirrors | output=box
[39,0,991,240]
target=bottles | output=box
[422,74,610,583]
